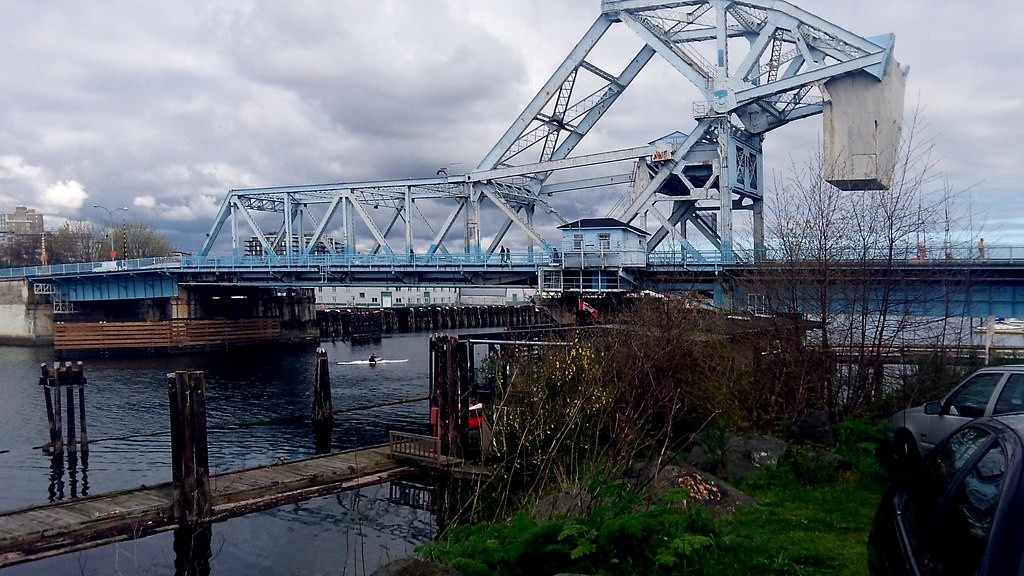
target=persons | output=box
[410,249,416,262]
[976,238,985,259]
[369,352,379,358]
[506,248,512,263]
[498,246,507,263]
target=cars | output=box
[885,364,1024,470]
[877,411,1023,576]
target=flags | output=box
[579,299,598,318]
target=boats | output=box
[973,317,1024,347]
[336,358,411,367]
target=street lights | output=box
[94,206,129,262]
